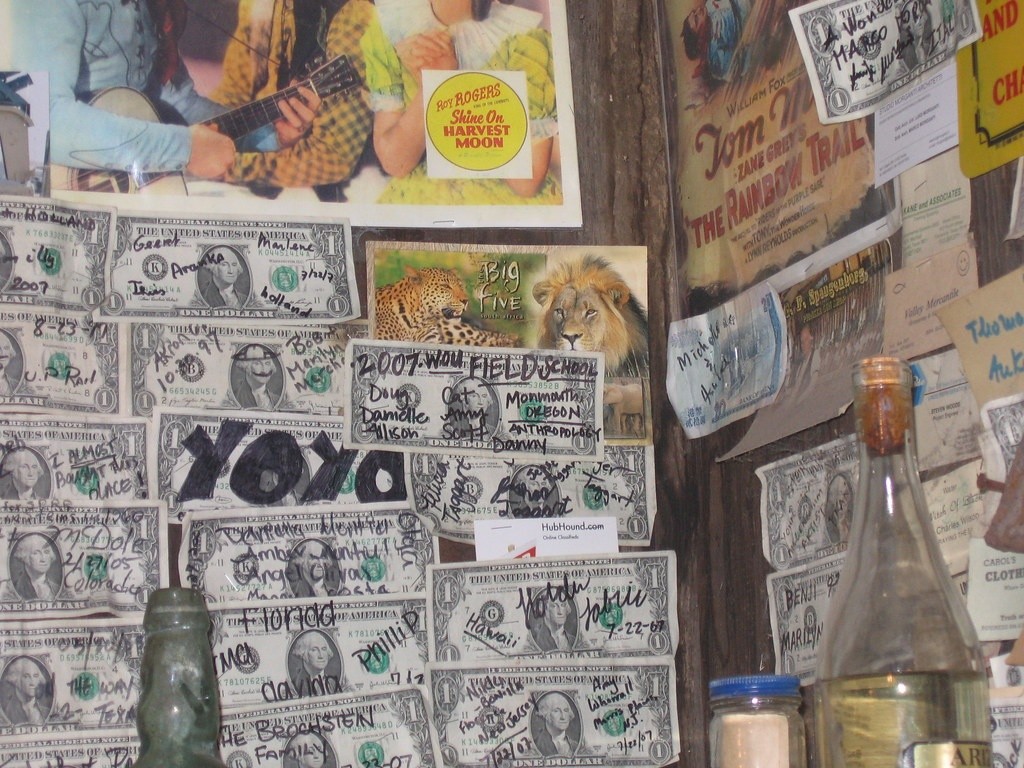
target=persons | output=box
[10,0,320,180]
[360,0,559,206]
[209,0,374,188]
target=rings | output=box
[296,123,304,132]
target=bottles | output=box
[130,587,228,768]
[708,675,808,768]
[810,356,991,768]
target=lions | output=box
[529,255,648,382]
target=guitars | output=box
[49,53,360,195]
